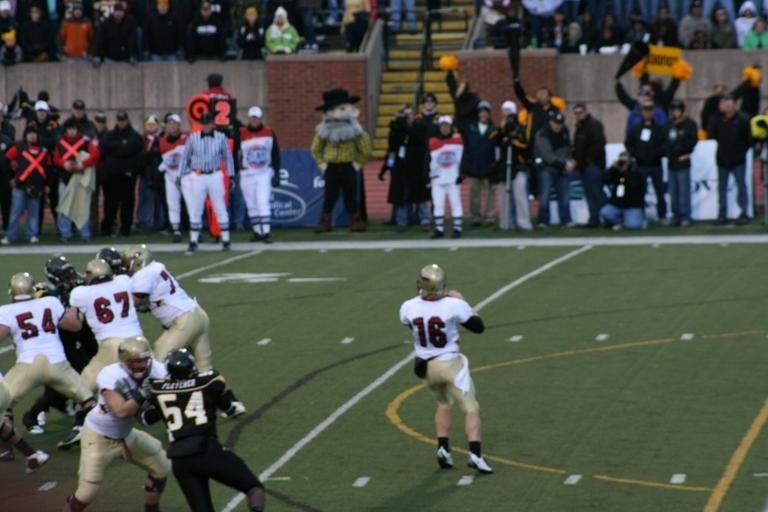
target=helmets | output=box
[9,243,151,298]
[165,347,199,379]
[416,264,446,292]
[118,336,152,364]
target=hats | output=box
[439,115,453,126]
[197,112,214,123]
[502,100,518,115]
[23,100,183,139]
[477,100,492,113]
[248,107,263,119]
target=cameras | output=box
[617,158,626,168]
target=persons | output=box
[397,261,495,475]
[0,244,266,511]
[1,1,768,255]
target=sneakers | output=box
[251,232,272,243]
[0,405,85,474]
[467,451,492,473]
[185,241,198,255]
[221,402,246,419]
[430,229,443,237]
[436,446,454,468]
